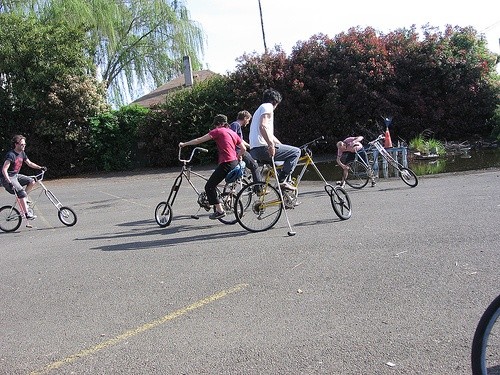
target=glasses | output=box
[18,144,26,146]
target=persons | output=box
[336,136,376,188]
[179,114,247,220]
[229,110,262,194]
[248,89,300,192]
[0,134,47,220]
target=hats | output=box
[212,114,227,126]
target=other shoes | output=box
[287,196,301,206]
[26,212,37,220]
[257,189,270,196]
[27,196,32,203]
[277,180,295,191]
[340,181,345,187]
[209,211,226,219]
[371,180,376,187]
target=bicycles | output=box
[154,136,352,233]
[472,294,500,374]
[0,169,77,234]
[342,131,418,190]
